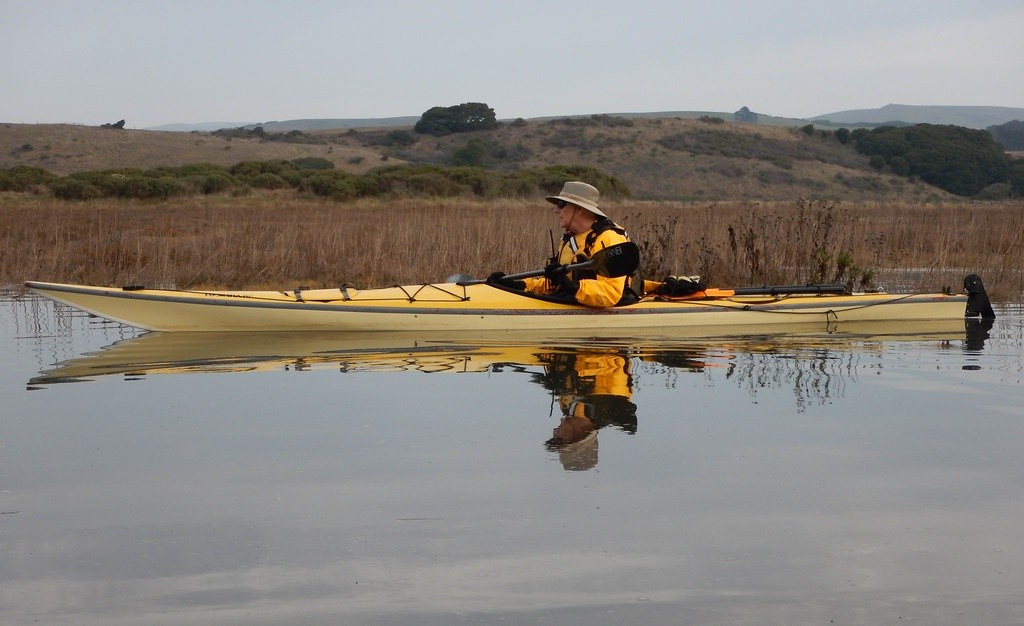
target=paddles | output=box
[641,278,847,300]
[443,237,646,289]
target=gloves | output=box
[487,272,514,290]
[545,264,570,285]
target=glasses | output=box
[556,200,572,209]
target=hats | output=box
[545,181,608,217]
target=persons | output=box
[535,350,638,469]
[487,181,643,308]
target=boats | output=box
[23,274,997,350]
